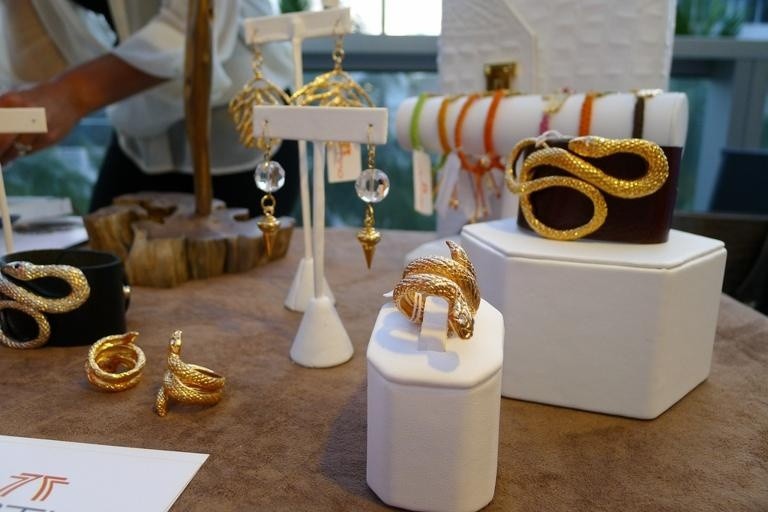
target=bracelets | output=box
[408,86,647,173]
[2,247,131,348]
[505,129,683,245]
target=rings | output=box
[156,330,226,416]
[394,240,480,339]
[12,140,34,156]
[85,331,146,391]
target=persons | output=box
[1,0,303,221]
